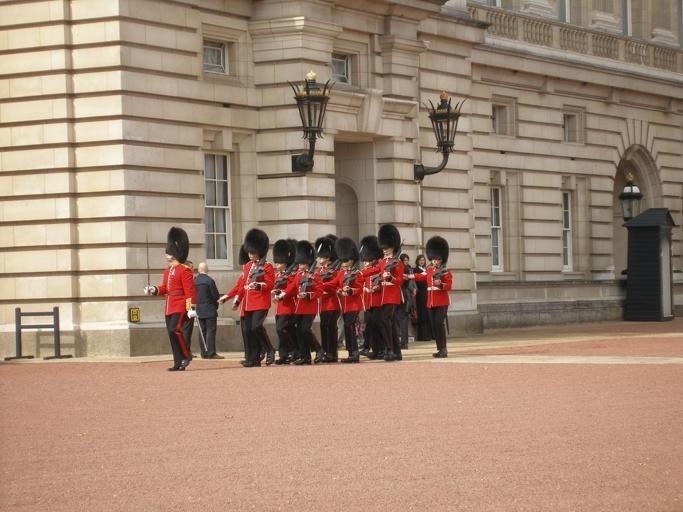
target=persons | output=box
[403,254,453,358]
[413,254,432,341]
[193,262,224,359]
[143,246,197,373]
[400,253,411,307]
[216,243,409,367]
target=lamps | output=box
[414,91,470,181]
[287,69,336,172]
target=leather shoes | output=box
[169,347,447,371]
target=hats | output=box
[166,227,189,263]
[239,225,449,267]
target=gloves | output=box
[187,309,197,319]
[144,286,156,297]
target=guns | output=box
[274,262,297,288]
[378,237,405,273]
[299,242,322,292]
[343,243,363,289]
[323,259,339,282]
[250,253,265,286]
[434,262,448,282]
[370,274,380,286]
